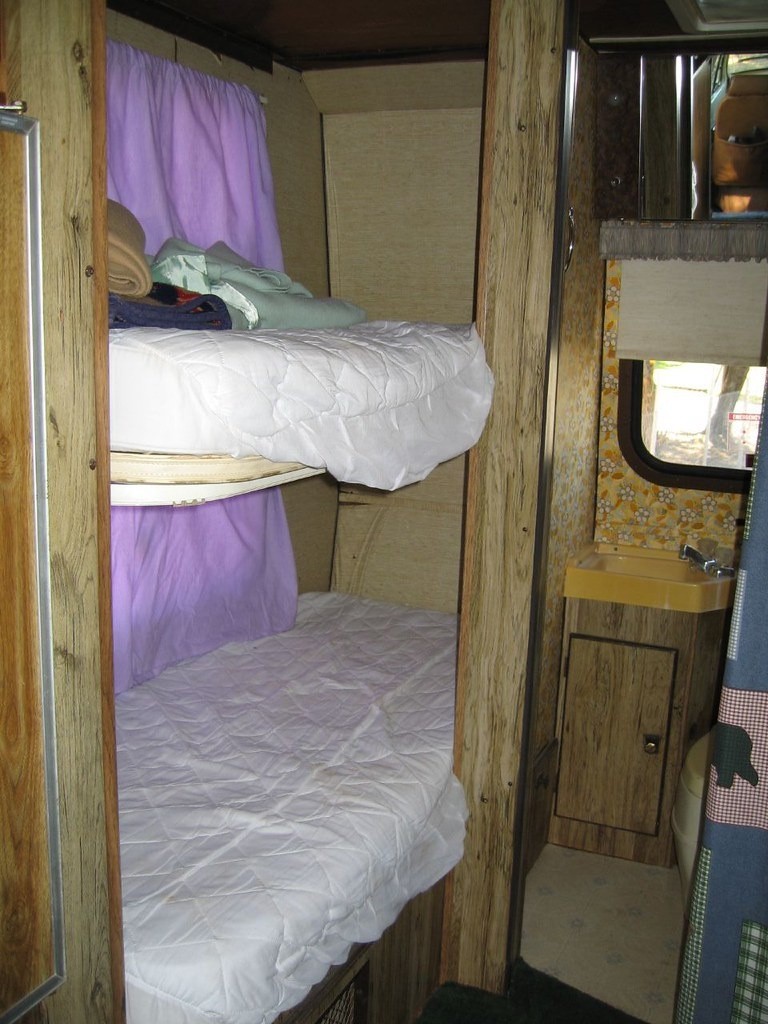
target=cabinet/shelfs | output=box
[546,594,728,874]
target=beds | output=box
[107,275,493,511]
[107,586,469,1024]
